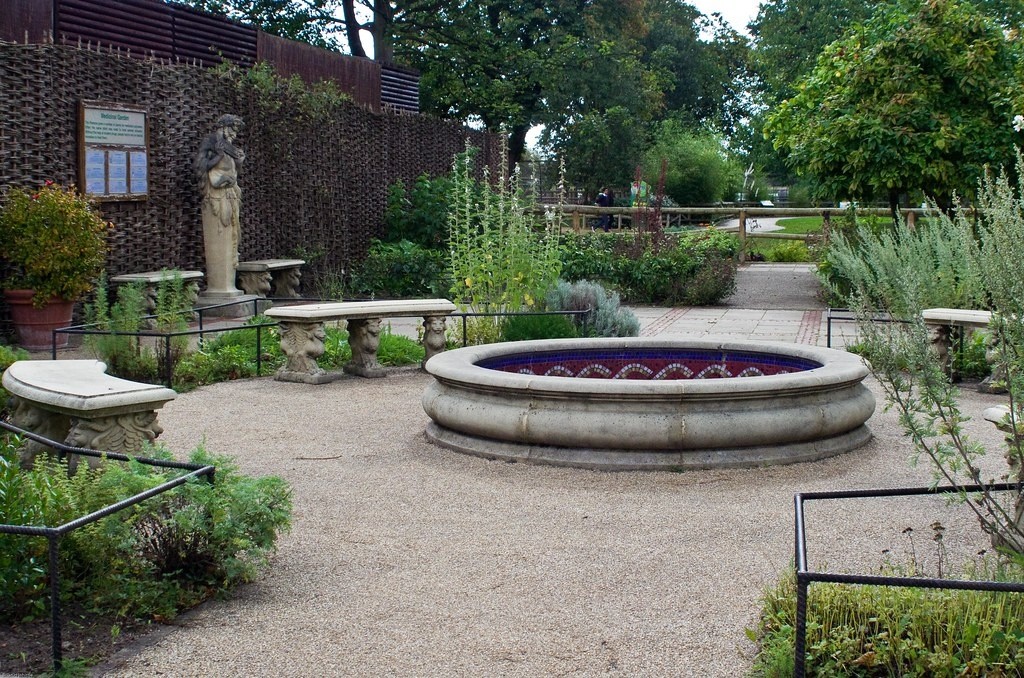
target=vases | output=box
[3,289,75,350]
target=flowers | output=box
[0,180,119,309]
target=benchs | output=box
[240,259,305,306]
[914,308,1024,393]
[1,358,177,474]
[112,269,205,328]
[264,298,458,385]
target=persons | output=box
[197,114,246,292]
[592,188,610,233]
